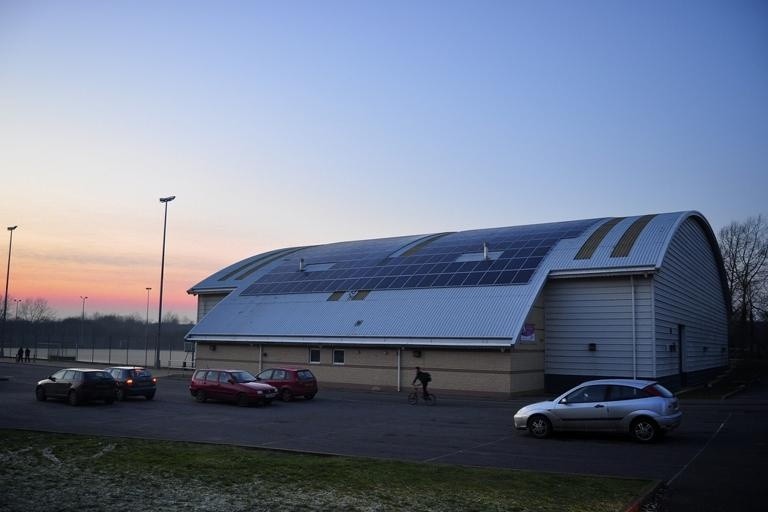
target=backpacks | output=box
[422,372,430,382]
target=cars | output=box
[512,376,683,444]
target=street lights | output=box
[157,194,177,369]
[144,287,152,366]
[0,226,19,381]
[13,298,22,318]
[79,296,89,344]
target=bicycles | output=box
[408,383,436,406]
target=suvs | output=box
[254,366,318,401]
[189,368,278,407]
[103,365,158,402]
[34,368,117,406]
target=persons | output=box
[411,366,428,399]
[25,347,30,362]
[18,347,23,362]
